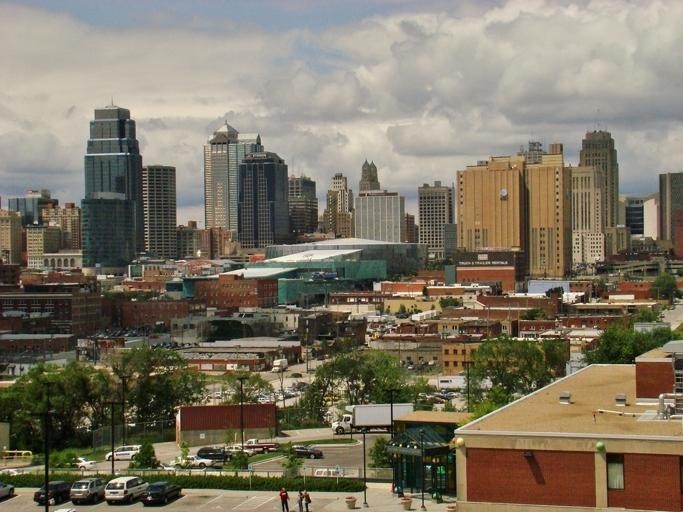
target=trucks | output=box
[272,358,288,373]
[332,403,413,434]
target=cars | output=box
[34,444,253,506]
[293,447,322,459]
[207,382,309,403]
[407,359,436,376]
[317,353,330,360]
[411,390,459,404]
[0,468,23,478]
[151,341,198,349]
[0,482,14,499]
[292,373,302,377]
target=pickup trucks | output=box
[240,438,279,454]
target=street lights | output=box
[419,429,426,511]
[26,381,57,512]
[108,375,137,474]
[236,376,249,448]
[361,427,368,507]
[382,388,402,438]
[462,360,474,412]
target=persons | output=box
[296,489,304,512]
[279,487,290,512]
[301,489,311,512]
